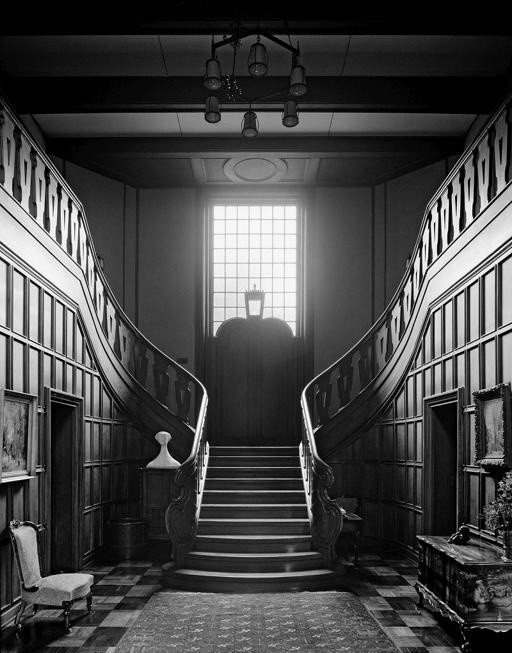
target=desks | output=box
[332,505,364,572]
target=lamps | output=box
[201,0,316,144]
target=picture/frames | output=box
[0,387,39,486]
[470,381,511,470]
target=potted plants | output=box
[474,469,512,563]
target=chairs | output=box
[6,516,96,632]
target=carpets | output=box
[108,587,404,653]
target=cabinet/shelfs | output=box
[411,531,512,652]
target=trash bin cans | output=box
[106,518,145,563]
